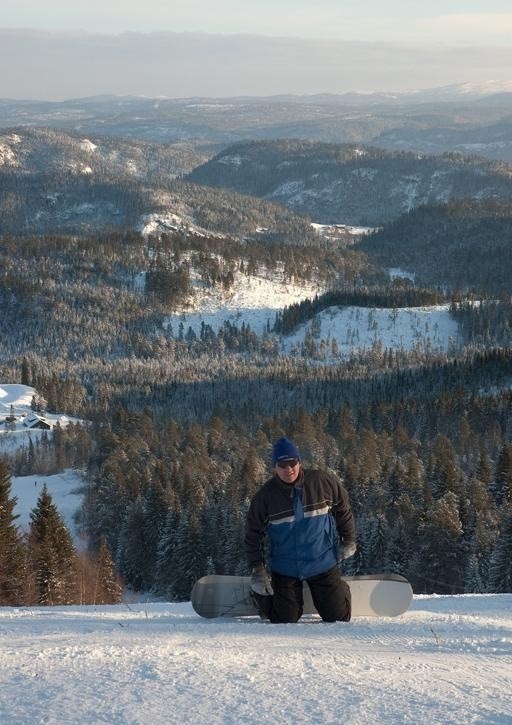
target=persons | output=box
[242,435,359,625]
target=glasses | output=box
[275,459,299,468]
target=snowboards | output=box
[190,575,413,619]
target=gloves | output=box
[337,542,356,562]
[250,566,274,596]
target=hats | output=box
[271,436,302,468]
